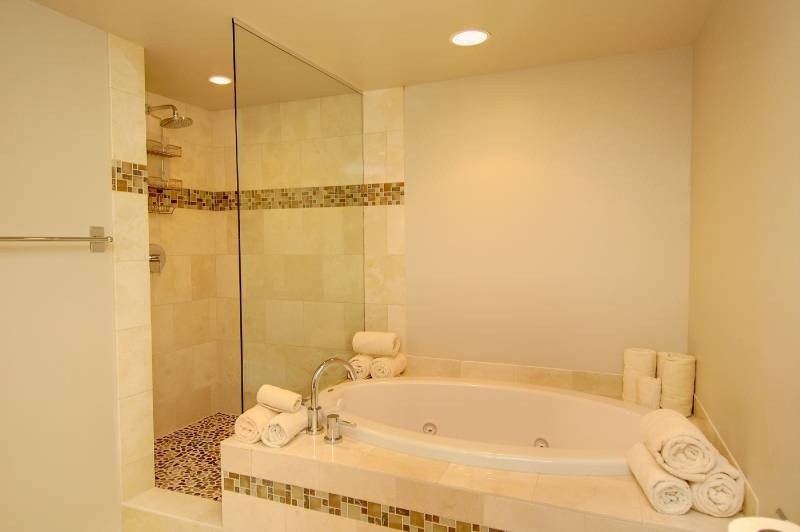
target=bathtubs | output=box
[315,376,650,477]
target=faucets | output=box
[305,357,357,436]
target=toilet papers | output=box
[727,516,800,532]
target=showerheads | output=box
[159,115,195,130]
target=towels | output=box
[624,408,747,518]
[234,383,308,450]
[345,331,408,381]
[621,347,697,419]
